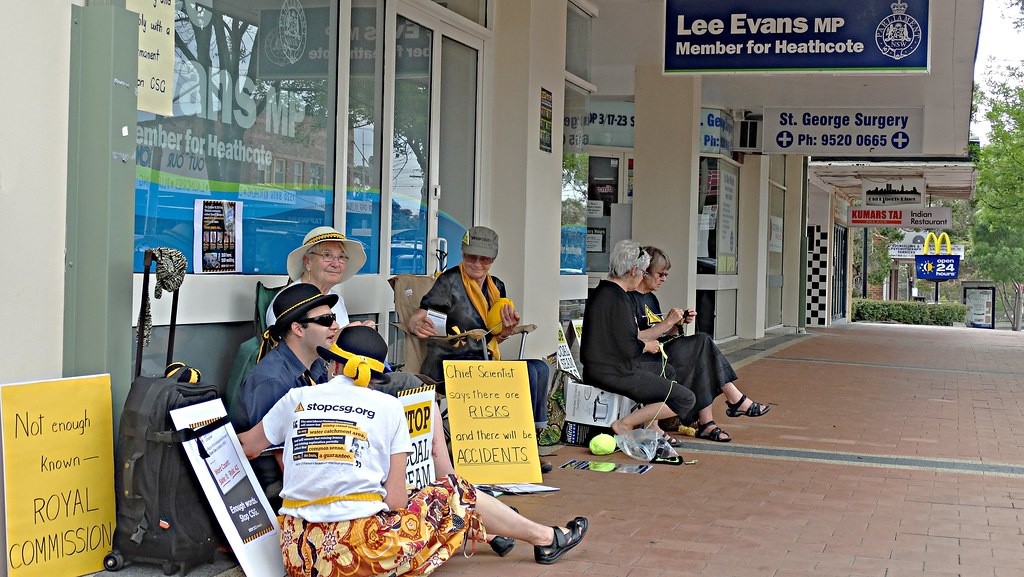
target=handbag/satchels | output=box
[419,334,493,396]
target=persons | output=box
[627,246,771,442]
[237,326,589,577]
[265,226,455,481]
[580,239,696,451]
[408,227,553,474]
[236,282,340,516]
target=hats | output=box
[256,282,340,363]
[316,326,391,387]
[462,226,498,259]
[286,226,367,284]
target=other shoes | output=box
[662,432,685,447]
[540,457,554,474]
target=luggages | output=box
[103,365,222,577]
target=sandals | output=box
[534,516,588,565]
[695,420,732,442]
[488,505,520,557]
[725,394,771,418]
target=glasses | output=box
[297,313,336,327]
[657,272,668,277]
[641,269,649,277]
[307,252,350,264]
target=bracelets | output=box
[498,334,512,340]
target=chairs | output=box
[388,273,536,447]
